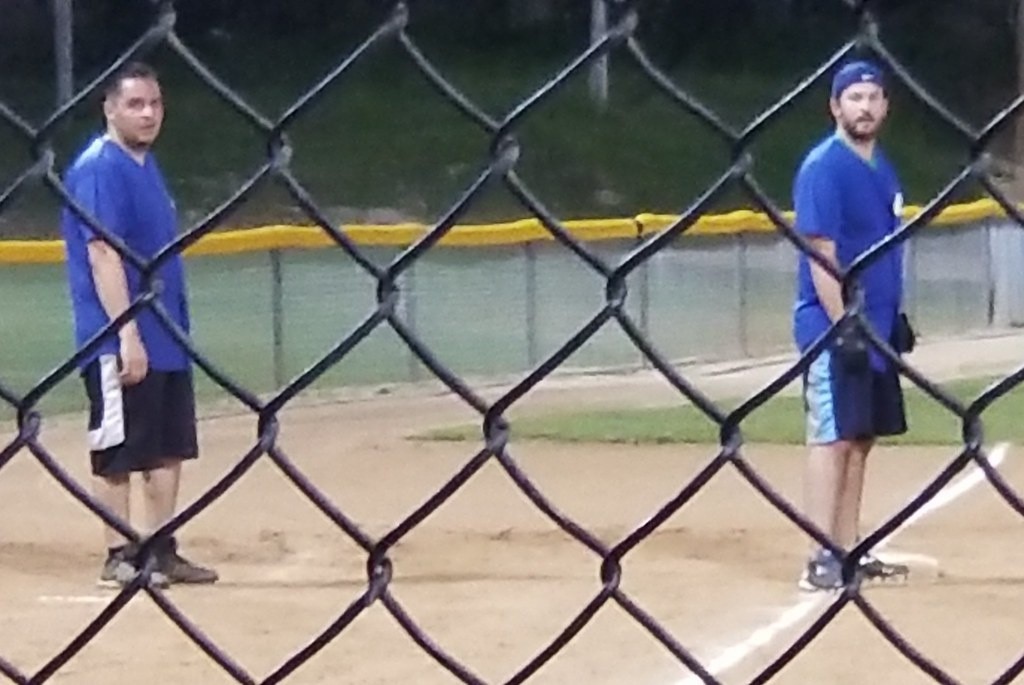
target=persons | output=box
[61,63,218,588]
[793,62,915,592]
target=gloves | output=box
[890,312,916,355]
[833,325,870,374]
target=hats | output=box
[831,61,887,100]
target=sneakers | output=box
[862,552,909,587]
[800,550,883,592]
[159,547,220,583]
[98,547,168,590]
